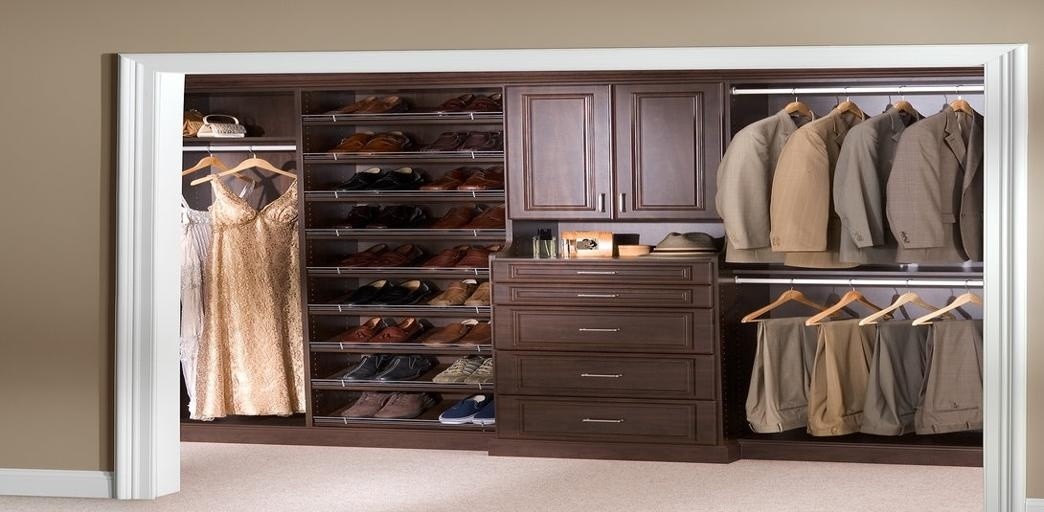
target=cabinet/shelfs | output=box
[501,74,770,227]
[295,86,506,428]
[489,252,766,446]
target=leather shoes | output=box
[352,316,492,344]
[344,278,490,306]
[336,206,505,229]
[338,243,501,267]
[343,355,450,381]
[420,130,502,152]
[341,392,436,419]
[328,131,409,154]
[341,95,405,114]
[432,93,502,116]
[332,167,504,190]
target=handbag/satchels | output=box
[185,108,246,139]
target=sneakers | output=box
[438,393,496,425]
[432,354,493,383]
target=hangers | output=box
[181,143,258,185]
[781,81,978,119]
[740,276,983,329]
[188,146,298,188]
